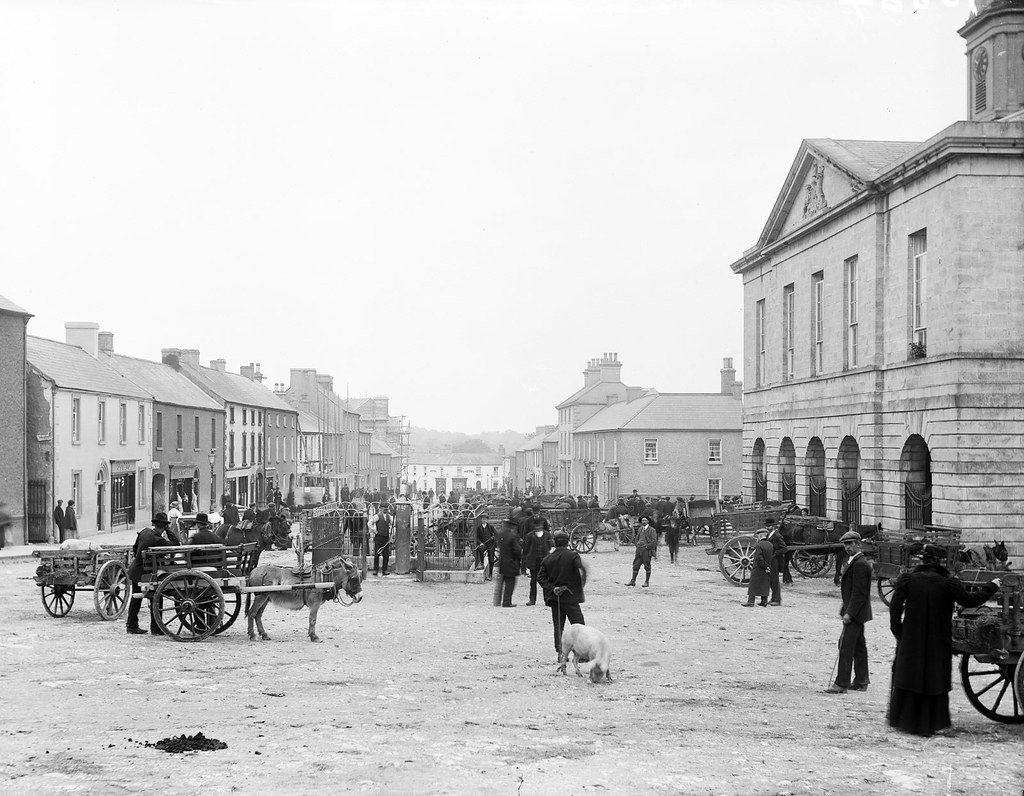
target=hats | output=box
[764,518,777,526]
[669,516,676,520]
[380,502,389,507]
[480,499,572,540]
[193,513,210,525]
[639,515,651,524]
[52,486,293,509]
[151,512,172,524]
[840,531,861,543]
[922,543,949,559]
[756,528,769,534]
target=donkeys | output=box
[247,557,364,644]
[594,514,634,552]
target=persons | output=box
[801,508,808,516]
[492,504,553,607]
[624,515,656,587]
[367,502,393,575]
[341,483,400,503]
[823,531,873,694]
[184,514,223,629]
[418,485,599,557]
[764,518,787,606]
[55,500,79,544]
[223,485,282,524]
[167,501,181,541]
[343,503,370,557]
[537,530,589,663]
[741,528,773,607]
[477,513,496,580]
[606,489,695,534]
[664,518,680,564]
[126,512,180,635]
[888,545,1001,737]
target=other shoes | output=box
[848,683,867,691]
[193,626,205,634]
[382,570,390,575]
[373,569,378,575]
[741,602,754,607]
[758,602,767,607]
[151,628,166,635]
[475,562,588,664]
[767,599,780,606]
[651,552,681,566]
[823,683,847,694]
[126,628,148,634]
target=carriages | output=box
[648,499,884,588]
[32,516,364,642]
[410,493,640,558]
[872,524,1024,723]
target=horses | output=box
[783,520,890,586]
[917,537,1010,572]
[215,519,293,572]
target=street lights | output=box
[208,450,216,515]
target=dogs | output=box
[559,623,612,684]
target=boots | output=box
[642,571,651,587]
[624,571,638,586]
